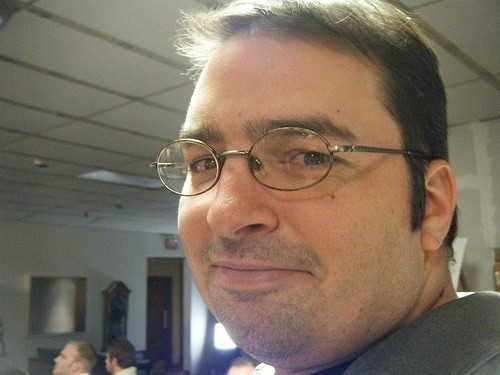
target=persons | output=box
[149,0,500,375]
[105,339,138,375]
[53,339,97,375]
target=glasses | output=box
[150,126,444,197]
